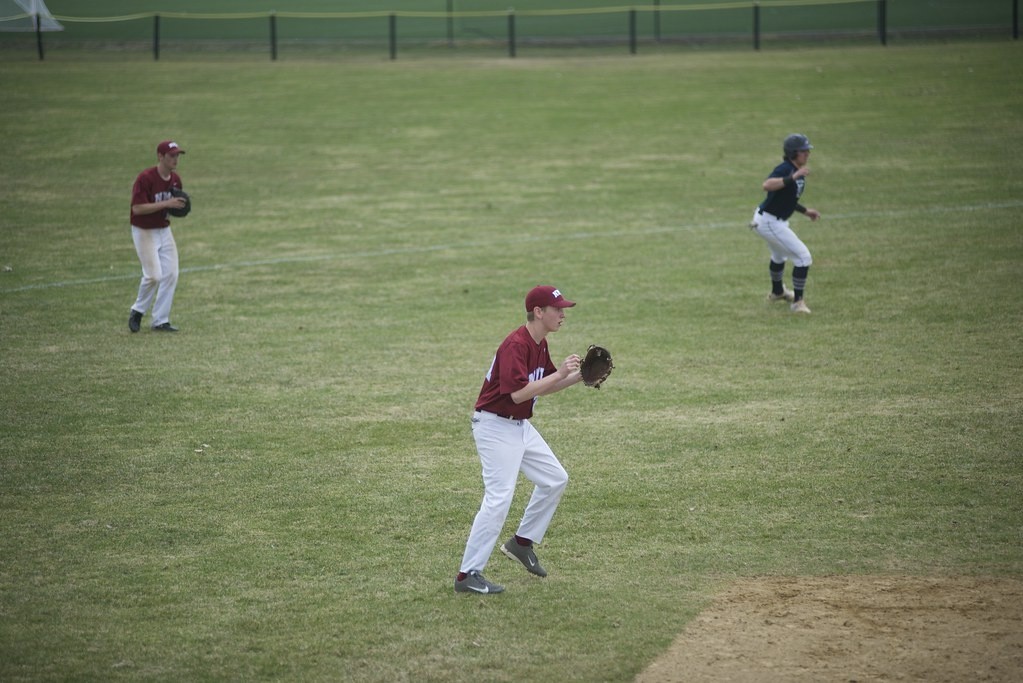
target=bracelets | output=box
[783,175,793,184]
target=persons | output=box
[751,133,821,314]
[454,285,614,594]
[128,141,191,332]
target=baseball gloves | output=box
[167,186,191,218]
[578,344,617,391]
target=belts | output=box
[475,407,521,421]
[758,209,781,219]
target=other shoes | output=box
[768,284,795,301]
[790,298,811,315]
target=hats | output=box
[157,141,186,156]
[783,134,813,151]
[524,284,577,310]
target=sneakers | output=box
[128,309,143,333]
[454,570,506,595]
[503,537,548,579]
[150,322,180,334]
